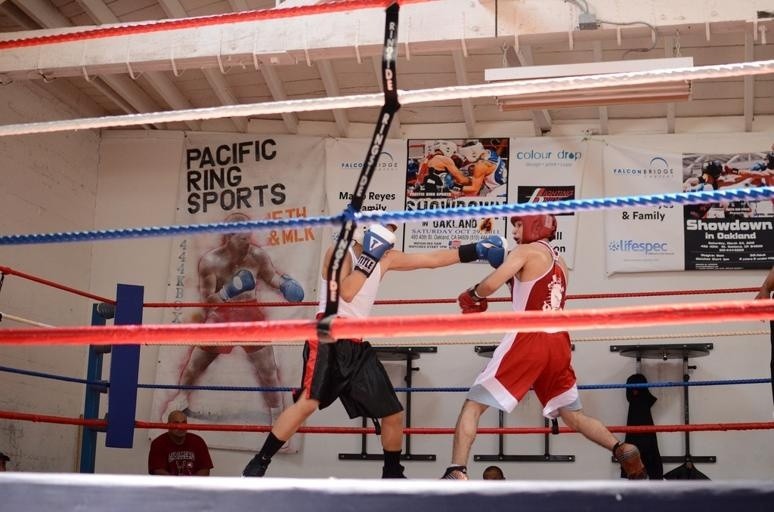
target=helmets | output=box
[702,159,723,181]
[434,140,457,158]
[511,215,557,244]
[460,139,485,164]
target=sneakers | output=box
[612,442,649,481]
[162,395,189,427]
[270,405,290,453]
[242,456,269,478]
[441,465,469,481]
[382,465,407,480]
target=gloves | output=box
[221,268,256,298]
[279,274,304,304]
[458,288,487,313]
[362,224,396,262]
[476,236,505,270]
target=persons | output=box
[160,213,304,453]
[413,140,472,198]
[243,219,509,479]
[449,141,507,196]
[483,466,506,480]
[684,160,764,219]
[148,411,215,476]
[0,453,10,472]
[721,152,774,187]
[442,213,648,480]
